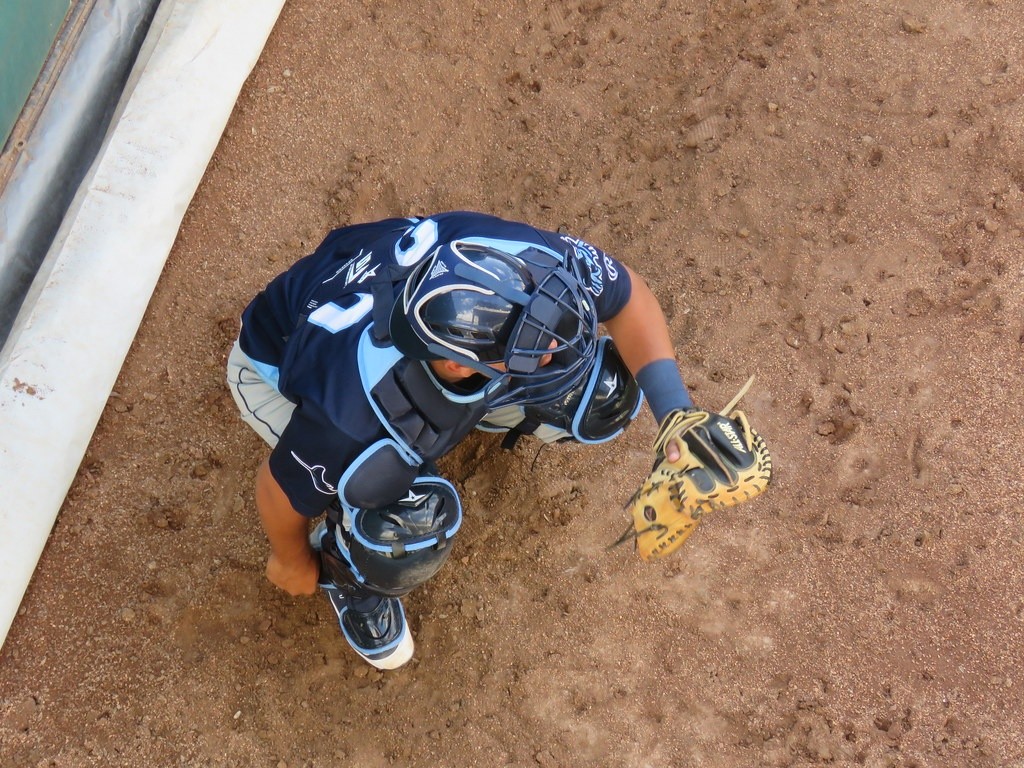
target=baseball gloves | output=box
[630,404,771,563]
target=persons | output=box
[226,211,771,671]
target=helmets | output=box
[386,244,533,366]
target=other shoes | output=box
[308,521,415,672]
